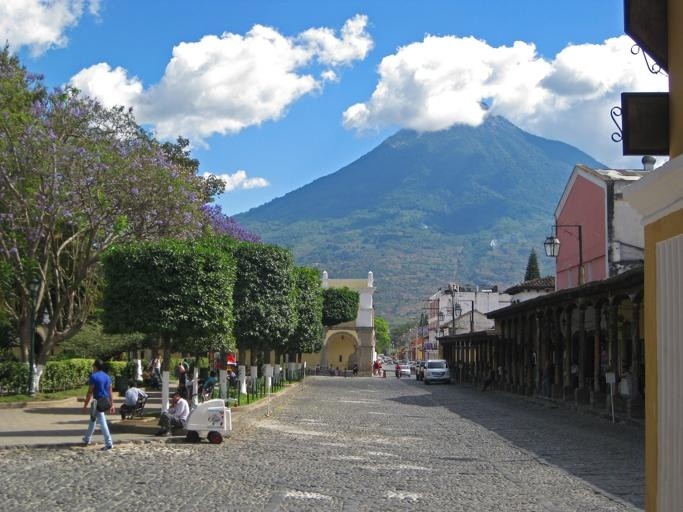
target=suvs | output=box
[414,359,424,381]
[422,359,451,385]
[397,365,411,378]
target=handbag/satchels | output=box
[96,398,112,412]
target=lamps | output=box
[542,221,583,267]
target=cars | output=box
[378,354,415,370]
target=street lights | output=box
[27,276,41,397]
[437,310,445,321]
[543,223,587,405]
[454,299,475,382]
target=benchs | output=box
[118,395,148,420]
[178,381,216,407]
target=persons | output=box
[352,361,359,376]
[179,358,237,393]
[120,381,149,420]
[395,363,401,369]
[142,353,162,391]
[82,358,116,451]
[155,392,190,435]
[542,361,552,401]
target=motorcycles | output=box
[395,368,401,378]
